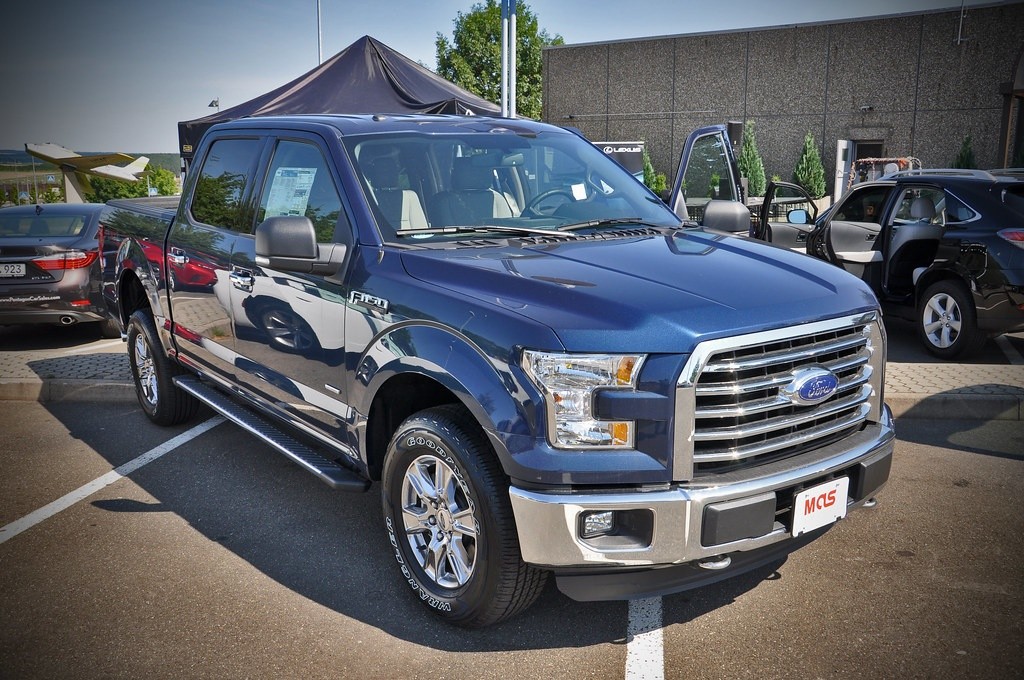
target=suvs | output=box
[755,168,1024,359]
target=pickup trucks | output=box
[98,113,897,629]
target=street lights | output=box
[208,97,219,113]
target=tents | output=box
[177,36,532,195]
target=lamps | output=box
[208,96,220,112]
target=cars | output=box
[1,203,122,339]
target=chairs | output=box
[30,219,49,234]
[427,155,513,228]
[332,143,427,244]
[884,196,943,296]
